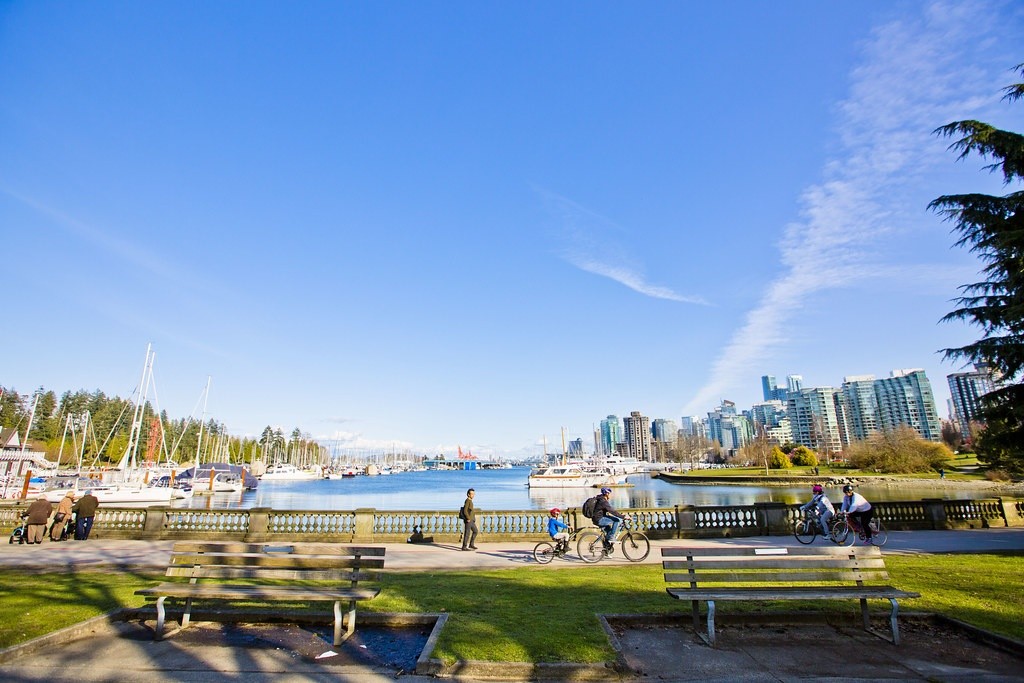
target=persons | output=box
[461,488,478,551]
[50,491,75,542]
[549,508,572,553]
[22,494,53,544]
[800,484,836,540]
[839,485,875,544]
[592,487,631,558]
[69,490,99,540]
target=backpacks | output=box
[582,494,605,519]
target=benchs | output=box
[661,547,920,648]
[131,543,387,644]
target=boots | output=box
[563,540,572,552]
[556,544,561,555]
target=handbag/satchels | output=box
[459,507,472,519]
[54,513,66,521]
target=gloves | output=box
[800,506,806,511]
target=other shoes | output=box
[864,537,874,545]
[462,548,471,551]
[76,537,81,540]
[36,541,42,544]
[604,554,612,559]
[27,542,34,544]
[55,539,61,541]
[83,537,87,540]
[608,537,622,544]
[822,534,830,539]
[469,546,478,549]
[51,538,55,541]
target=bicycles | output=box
[832,510,888,547]
[533,522,577,564]
[794,507,849,545]
[576,512,651,564]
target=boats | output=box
[527,422,629,489]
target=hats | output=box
[601,487,612,496]
[85,489,92,495]
[550,508,560,515]
[813,486,822,492]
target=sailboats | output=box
[0,342,430,509]
